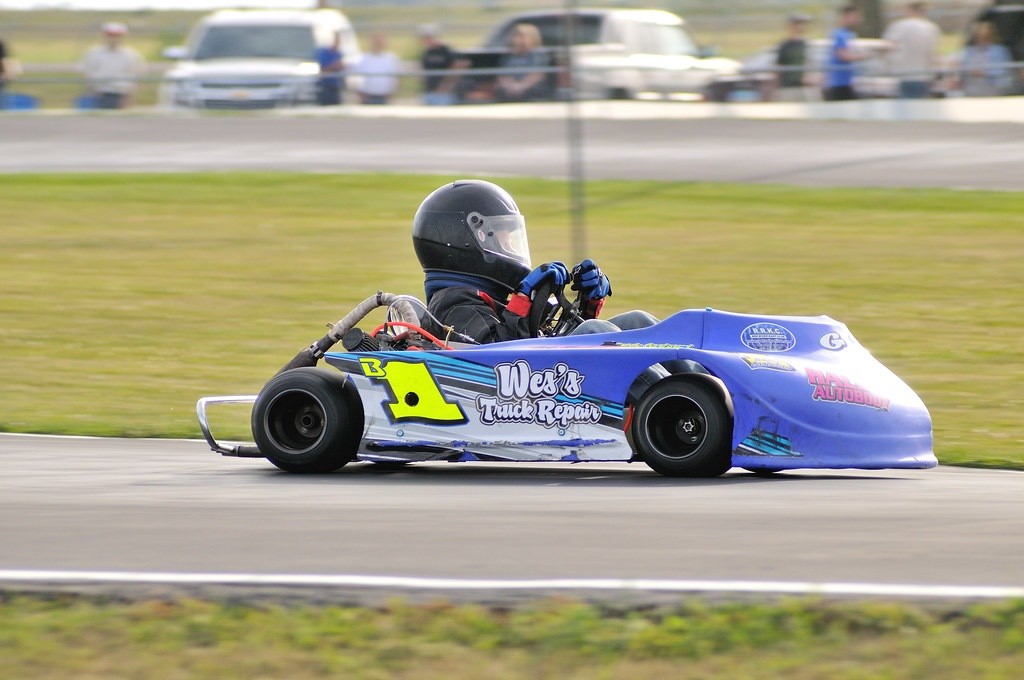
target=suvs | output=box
[154,9,366,103]
[946,0,1024,97]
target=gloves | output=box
[570,258,612,302]
[515,260,573,297]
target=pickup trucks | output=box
[426,7,745,100]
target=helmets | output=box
[411,179,532,289]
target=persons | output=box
[821,5,889,100]
[81,22,142,107]
[883,1,940,98]
[762,11,812,101]
[489,24,551,101]
[311,33,343,104]
[417,23,451,90]
[936,18,1010,97]
[358,35,398,103]
[413,180,661,344]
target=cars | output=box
[705,41,951,101]
